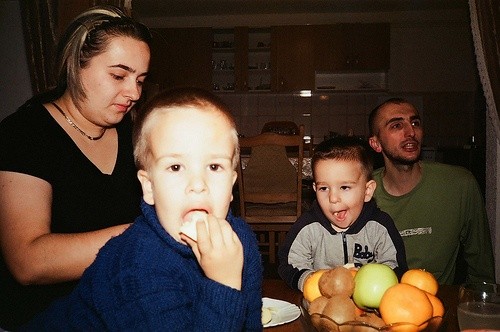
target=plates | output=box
[262,297,301,328]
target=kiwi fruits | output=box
[309,267,386,332]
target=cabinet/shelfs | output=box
[149,22,391,93]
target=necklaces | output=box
[52,101,106,140]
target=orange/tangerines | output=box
[380,269,444,332]
[303,268,362,317]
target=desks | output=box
[261,277,500,332]
[240,135,326,177]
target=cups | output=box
[456,282,500,330]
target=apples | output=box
[352,263,399,311]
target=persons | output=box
[0,5,151,332]
[32,96,263,332]
[277,138,407,293]
[369,98,494,287]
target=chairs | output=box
[237,124,307,265]
